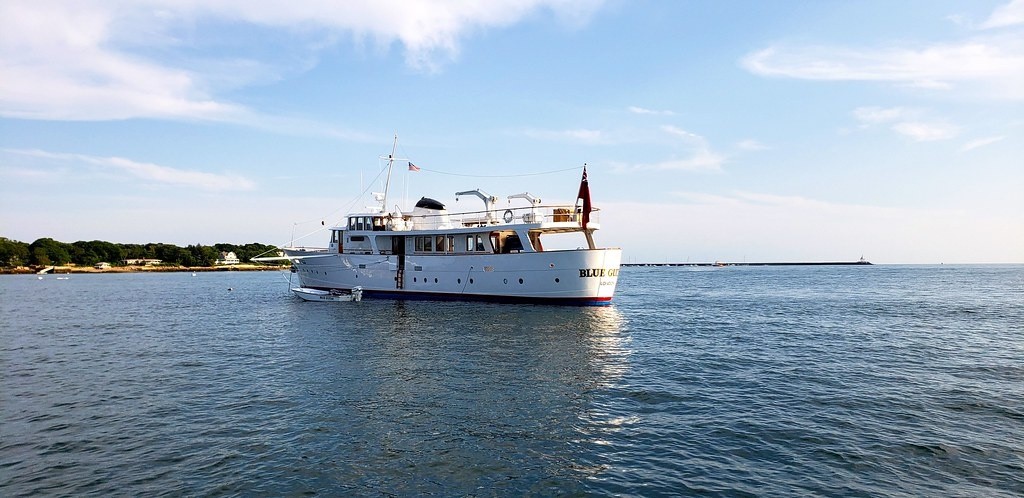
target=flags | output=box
[578,163,592,230]
[409,162,420,172]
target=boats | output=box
[290,286,364,302]
[247,131,623,306]
